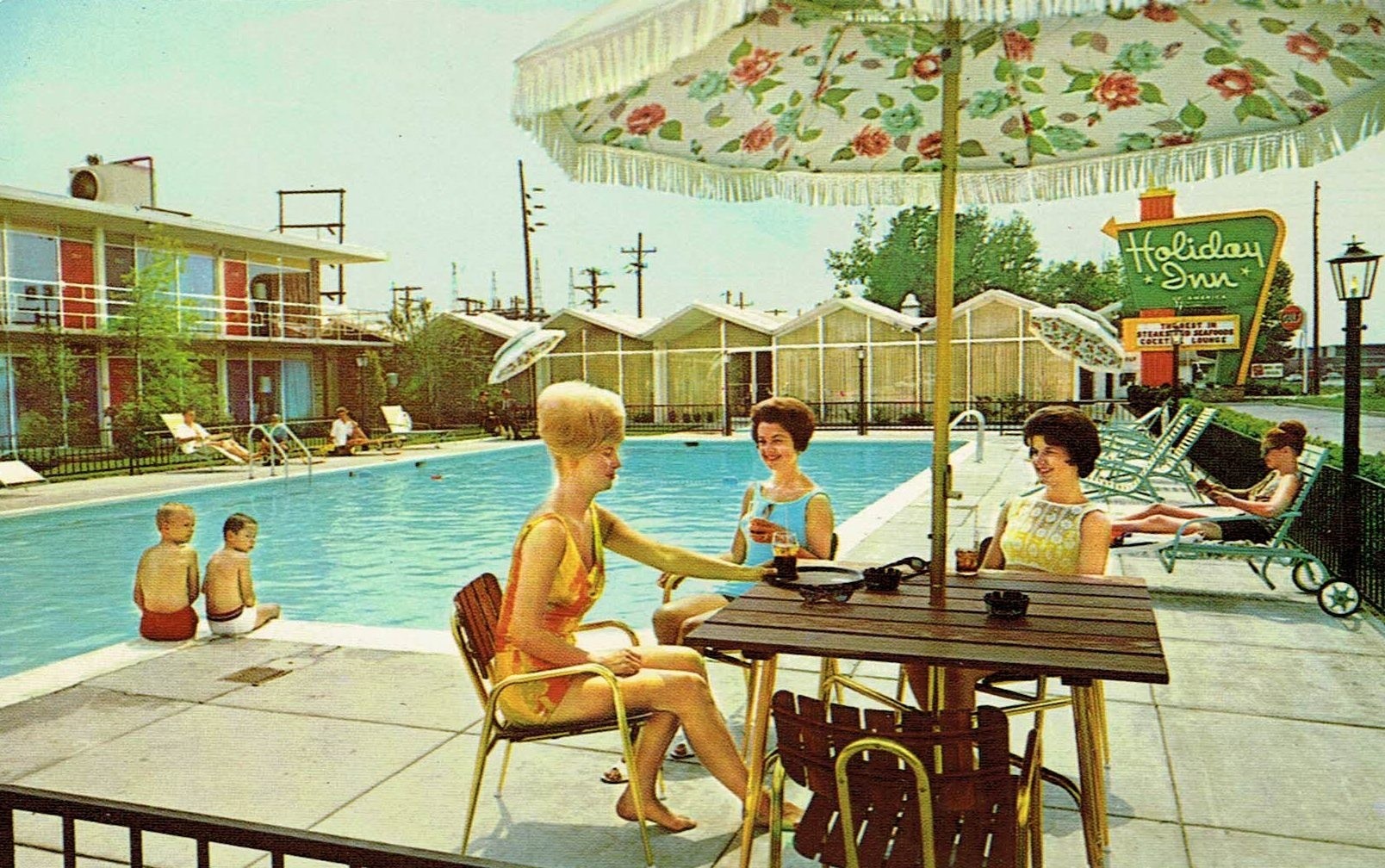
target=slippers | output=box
[670,739,696,759]
[600,759,630,784]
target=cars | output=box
[1321,372,1343,382]
[1284,374,1303,382]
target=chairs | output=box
[450,573,665,867]
[661,530,839,763]
[380,406,457,449]
[1021,403,1361,618]
[769,689,1045,868]
[160,413,256,472]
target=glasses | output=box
[880,557,932,581]
[1261,445,1280,457]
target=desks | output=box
[683,558,1170,868]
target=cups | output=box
[952,529,979,576]
[773,531,800,581]
[748,501,770,535]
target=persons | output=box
[261,413,289,466]
[904,406,1111,711]
[133,501,202,641]
[174,405,263,461]
[201,512,280,636]
[329,404,379,455]
[1109,420,1308,545]
[475,389,521,440]
[491,381,805,832]
[599,398,832,782]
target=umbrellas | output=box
[514,0,1385,606]
